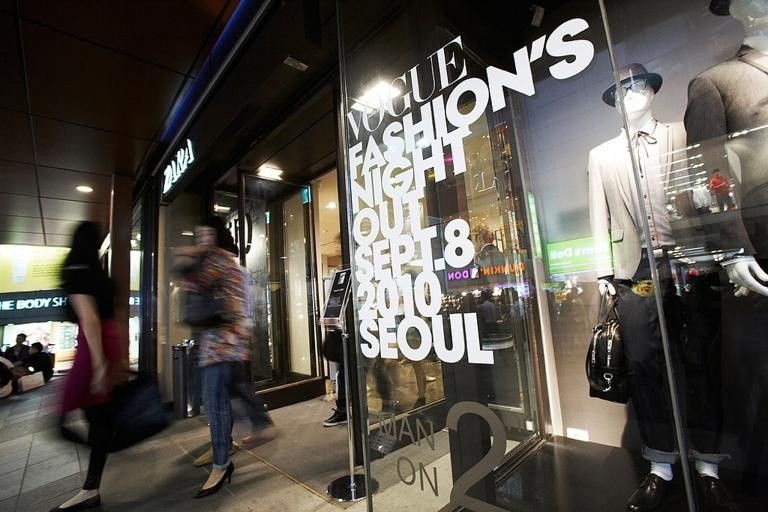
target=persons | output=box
[167,218,251,499]
[470,225,503,265]
[48,220,129,512]
[321,231,393,427]
[455,286,583,342]
[384,268,427,409]
[583,62,734,512]
[710,167,732,212]
[0,333,54,400]
[193,262,278,467]
[682,0,768,511]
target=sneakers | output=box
[322,408,347,428]
[242,426,279,443]
[193,444,234,467]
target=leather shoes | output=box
[694,468,738,512]
[49,494,100,512]
[624,472,677,512]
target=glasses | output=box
[610,77,648,101]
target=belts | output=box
[639,247,677,262]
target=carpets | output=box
[198,393,451,510]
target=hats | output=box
[602,63,661,108]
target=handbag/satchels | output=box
[584,318,630,405]
[321,331,343,365]
[180,286,235,330]
[83,371,166,453]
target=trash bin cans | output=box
[172,339,200,419]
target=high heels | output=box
[195,459,234,500]
